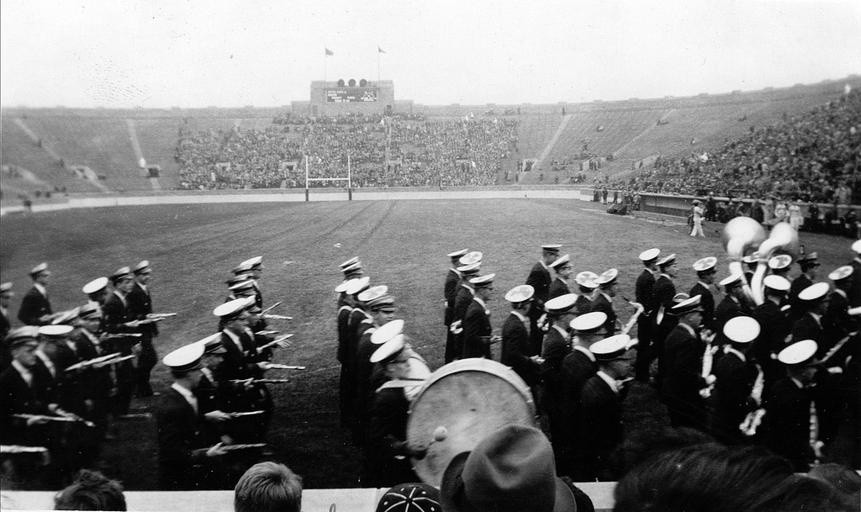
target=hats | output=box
[163,256,264,372]
[693,257,747,288]
[638,248,679,267]
[375,424,576,512]
[0,282,15,298]
[4,301,102,347]
[723,316,761,343]
[447,249,495,285]
[764,239,861,301]
[671,293,704,315]
[29,263,50,278]
[82,260,152,296]
[778,339,818,367]
[504,245,640,361]
[335,256,405,365]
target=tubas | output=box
[722,216,800,306]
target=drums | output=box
[406,359,538,490]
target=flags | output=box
[325,48,334,56]
[378,47,387,53]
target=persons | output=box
[0,241,861,512]
[590,88,860,238]
[173,105,611,188]
